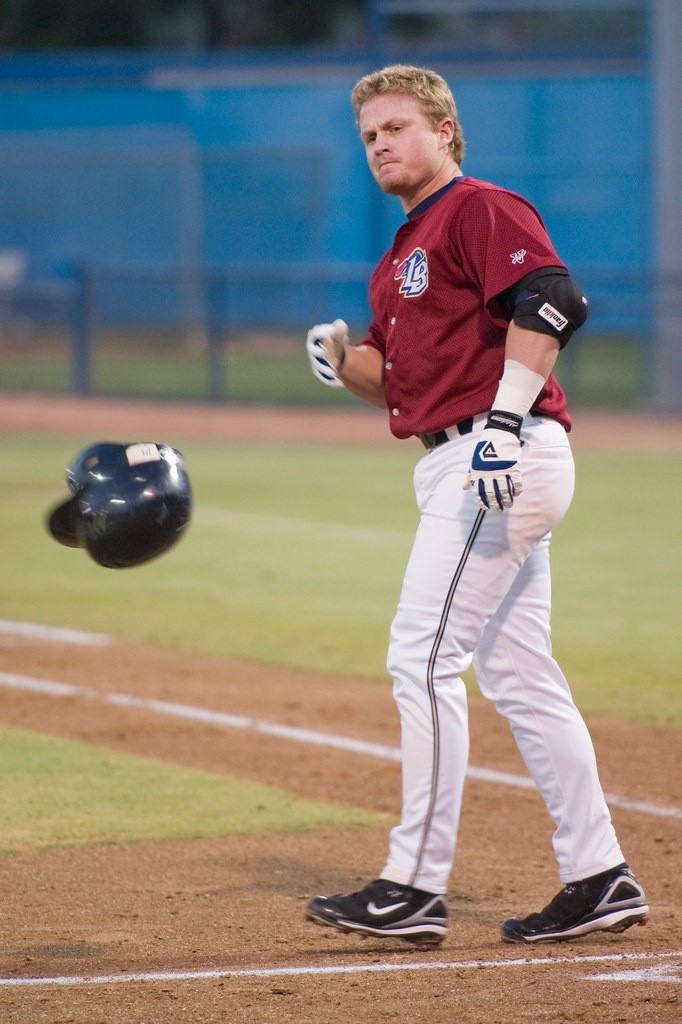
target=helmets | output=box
[46,442,195,570]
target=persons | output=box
[298,65,652,948]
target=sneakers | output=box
[500,864,649,946]
[306,878,454,953]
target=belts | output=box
[416,419,477,451]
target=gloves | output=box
[305,318,350,391]
[463,410,524,514]
